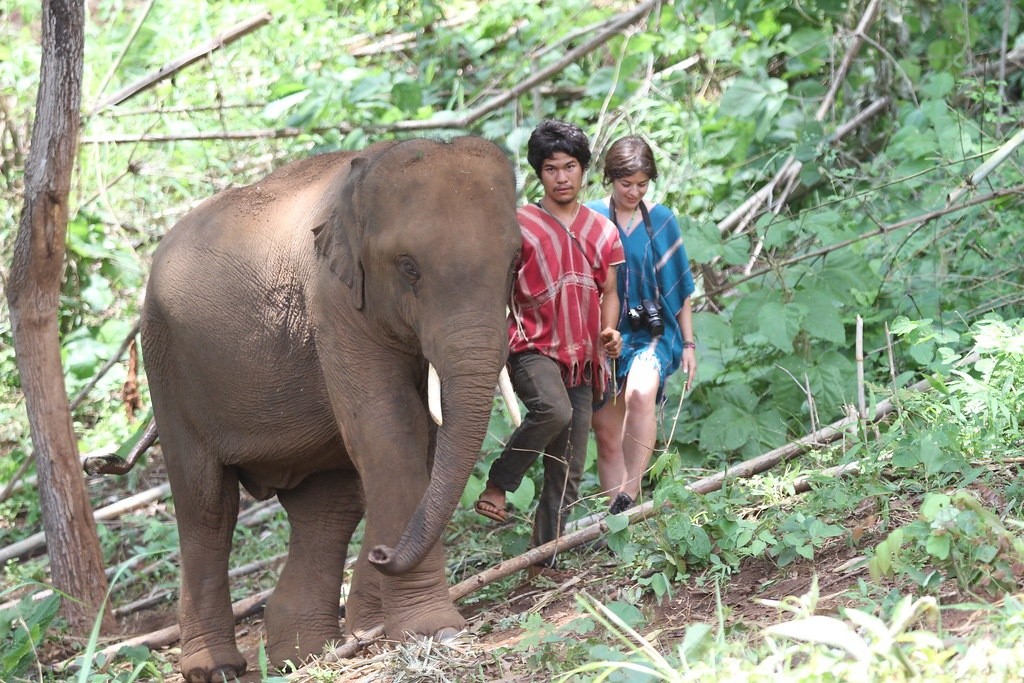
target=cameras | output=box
[627,298,665,335]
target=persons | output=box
[475,119,624,584]
[585,135,698,517]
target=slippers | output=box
[473,499,506,524]
[529,569,571,581]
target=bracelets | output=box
[683,342,696,351]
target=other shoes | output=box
[598,492,635,523]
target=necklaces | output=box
[624,206,637,235]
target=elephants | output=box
[140,136,524,683]
